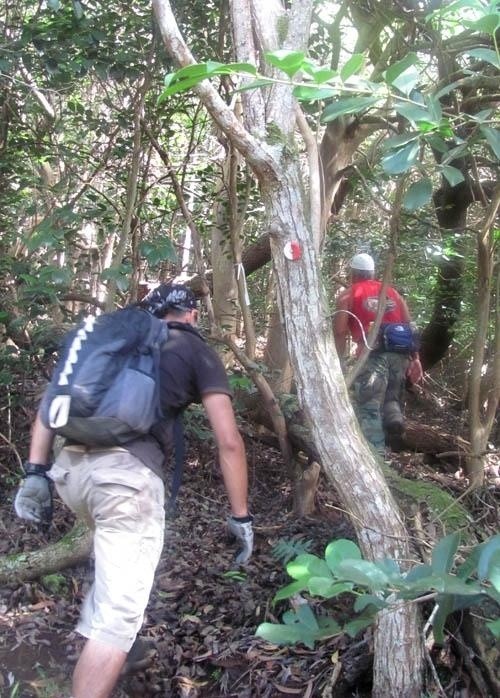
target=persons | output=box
[13,283,254,697]
[332,253,424,458]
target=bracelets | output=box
[25,462,50,474]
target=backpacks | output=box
[38,310,203,448]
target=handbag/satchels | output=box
[380,322,422,355]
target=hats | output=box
[348,253,377,272]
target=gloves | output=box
[15,464,54,528]
[226,514,256,564]
